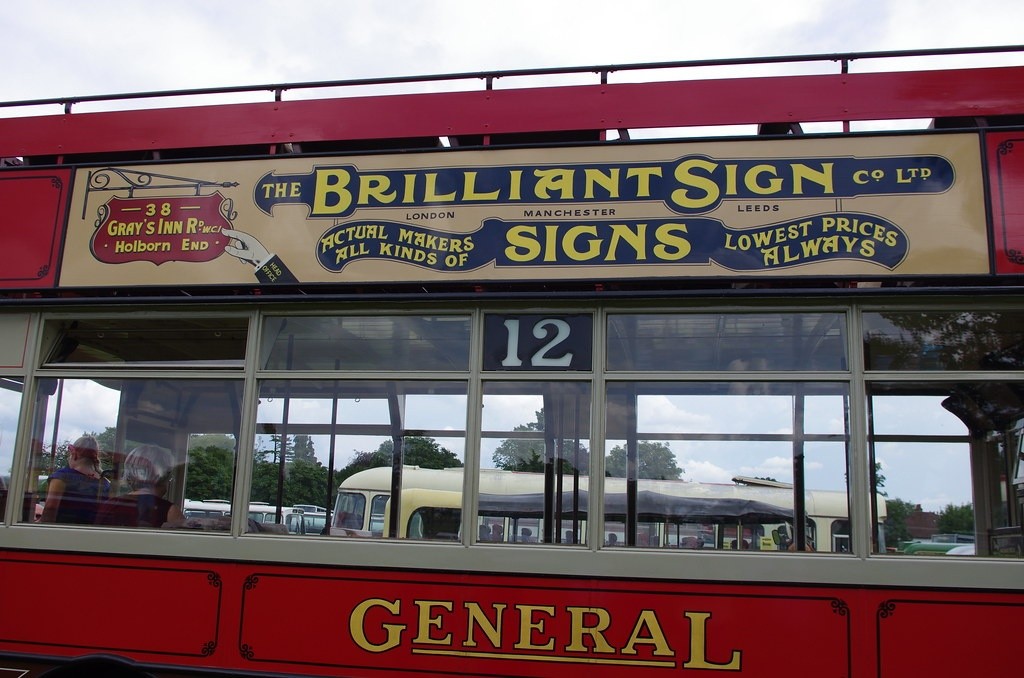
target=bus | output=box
[332,462,890,557]
[899,540,976,554]
[0,46,1024,678]
[183,497,334,534]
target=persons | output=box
[34,434,114,527]
[93,445,185,529]
[640,533,750,551]
[564,529,573,544]
[477,524,503,542]
[520,527,532,543]
[607,533,618,546]
